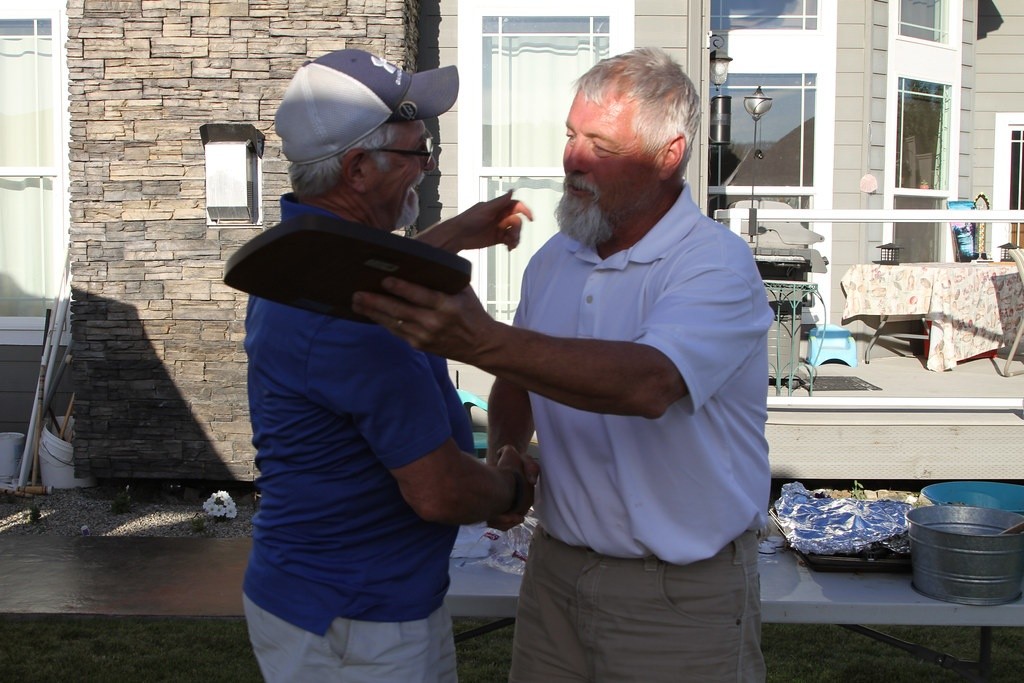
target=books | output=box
[222,211,473,329]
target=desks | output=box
[841,262,1024,372]
[448,517,1024,683]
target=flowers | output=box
[202,488,237,520]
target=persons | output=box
[239,48,538,682]
[352,44,776,683]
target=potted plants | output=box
[920,179,930,189]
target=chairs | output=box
[1004,248,1024,376]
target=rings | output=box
[386,319,404,329]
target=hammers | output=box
[17,354,55,495]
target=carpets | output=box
[801,376,883,391]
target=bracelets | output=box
[492,470,527,517]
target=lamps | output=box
[742,85,767,243]
[712,32,732,92]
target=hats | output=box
[275,48,459,166]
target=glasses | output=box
[342,128,434,168]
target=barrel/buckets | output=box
[905,506,1024,605]
[921,482,1024,513]
[39,416,95,489]
[0,432,26,484]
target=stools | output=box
[762,280,827,396]
[806,324,858,369]
